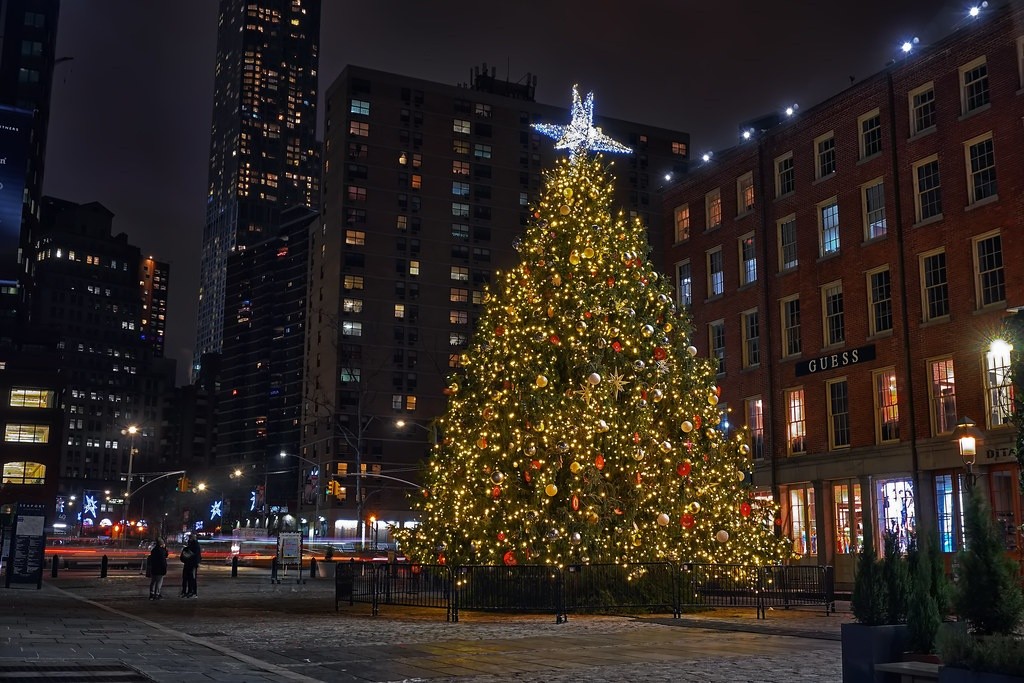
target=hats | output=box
[155,536,164,545]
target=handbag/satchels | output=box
[151,562,167,576]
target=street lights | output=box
[955,417,979,505]
[234,469,267,526]
[120,425,138,541]
[198,482,223,522]
[279,450,321,539]
[394,419,435,440]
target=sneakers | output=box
[188,594,198,599]
[178,592,192,599]
[149,594,163,600]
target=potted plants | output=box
[841,512,954,683]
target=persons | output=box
[180,534,201,599]
[149,537,168,598]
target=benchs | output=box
[874,661,944,683]
